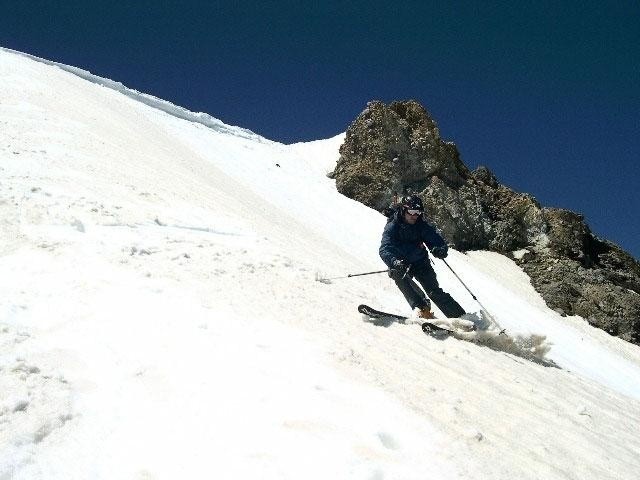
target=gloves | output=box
[433,247,448,259]
[393,259,408,278]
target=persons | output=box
[379,195,468,321]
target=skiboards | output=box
[358,304,559,368]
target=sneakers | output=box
[417,299,431,319]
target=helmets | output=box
[402,196,425,210]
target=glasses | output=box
[407,209,422,216]
